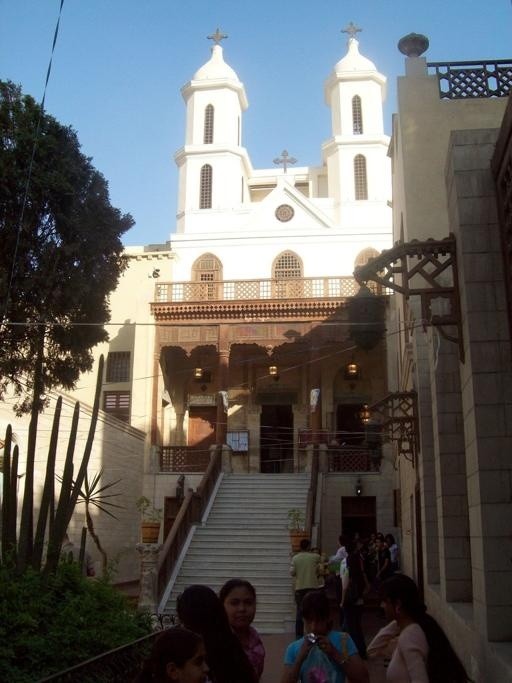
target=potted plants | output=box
[282,504,311,551]
[135,494,166,544]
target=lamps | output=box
[359,404,372,423]
[343,360,362,381]
[267,363,281,382]
[354,476,362,496]
[345,228,467,368]
[192,364,203,379]
[364,413,416,469]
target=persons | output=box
[218,579,265,683]
[279,591,369,683]
[288,528,400,662]
[365,574,467,683]
[176,585,259,683]
[131,626,211,683]
